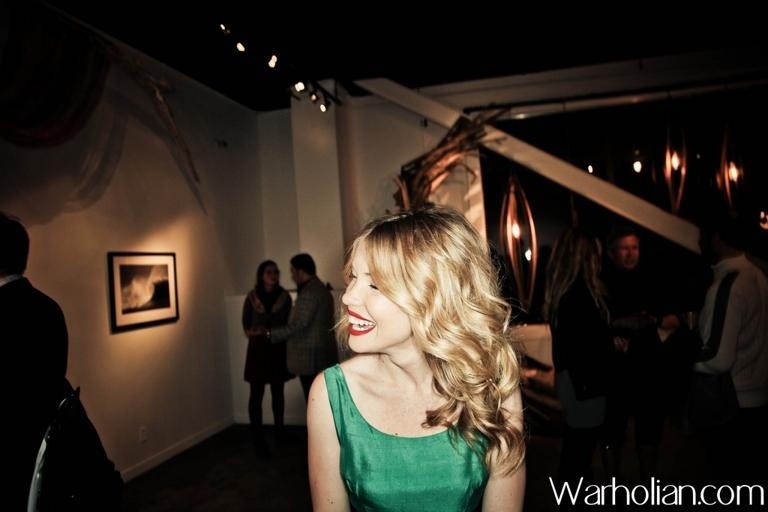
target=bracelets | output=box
[265,329,272,339]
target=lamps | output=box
[220,19,342,112]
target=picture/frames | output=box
[107,251,179,334]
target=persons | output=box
[240,259,292,440]
[1,215,68,511]
[548,209,768,511]
[303,207,530,511]
[245,252,338,402]
